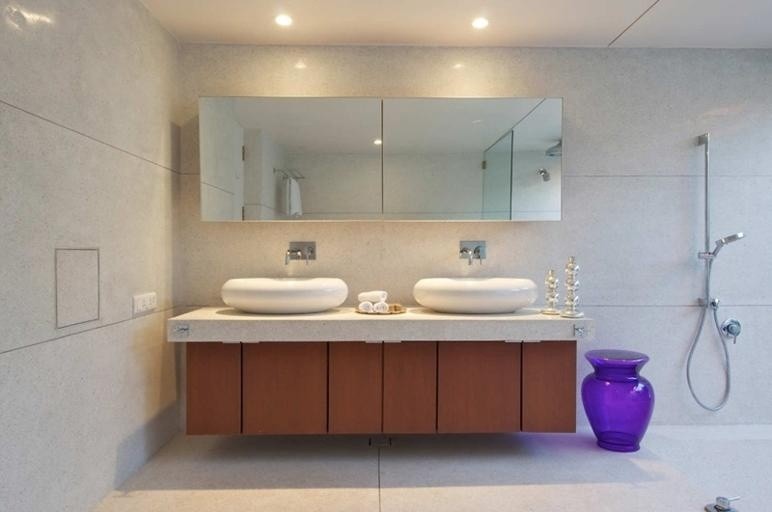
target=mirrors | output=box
[199,97,565,222]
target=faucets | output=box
[459,245,485,267]
[284,246,315,266]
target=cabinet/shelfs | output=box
[165,340,593,434]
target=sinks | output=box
[221,276,350,314]
[411,275,540,314]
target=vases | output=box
[581,349,656,454]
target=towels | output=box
[391,304,406,313]
[374,303,389,314]
[357,290,388,302]
[281,177,302,217]
[359,302,373,313]
